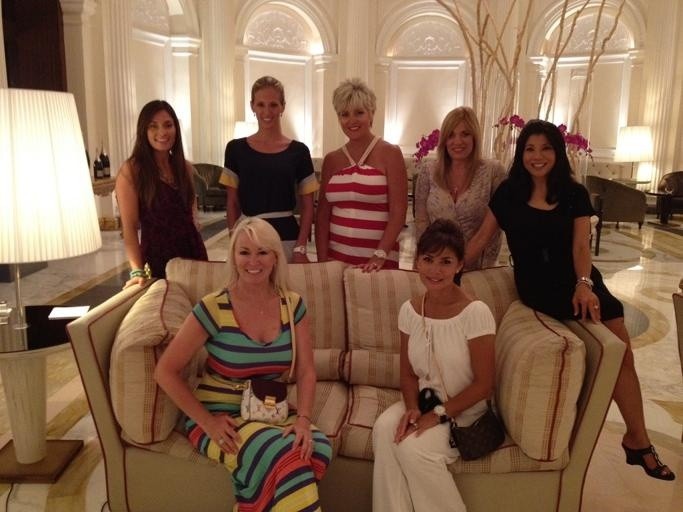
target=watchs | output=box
[432,404,447,424]
[373,249,388,260]
[292,245,307,255]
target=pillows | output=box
[108,278,208,442]
[490,298,589,463]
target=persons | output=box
[313,76,408,275]
[216,75,321,264]
[114,99,209,291]
[413,105,506,270]
[151,214,334,512]
[464,118,678,481]
[369,216,500,510]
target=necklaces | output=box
[444,161,476,203]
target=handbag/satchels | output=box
[450,409,505,461]
[241,379,288,423]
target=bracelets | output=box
[296,413,312,424]
[575,276,595,291]
[130,269,148,278]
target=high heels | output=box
[621,443,675,480]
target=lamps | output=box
[612,124,655,184]
[0,87,100,330]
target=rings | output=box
[593,305,600,310]
[308,439,314,444]
[413,423,418,429]
[217,438,224,445]
[372,263,377,268]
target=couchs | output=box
[65,255,628,512]
[190,162,229,211]
[655,171,682,224]
[584,174,647,231]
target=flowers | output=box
[491,111,524,166]
[557,124,593,173]
[407,128,440,173]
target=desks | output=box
[0,306,92,483]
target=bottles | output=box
[100,143,110,178]
[93,146,104,181]
